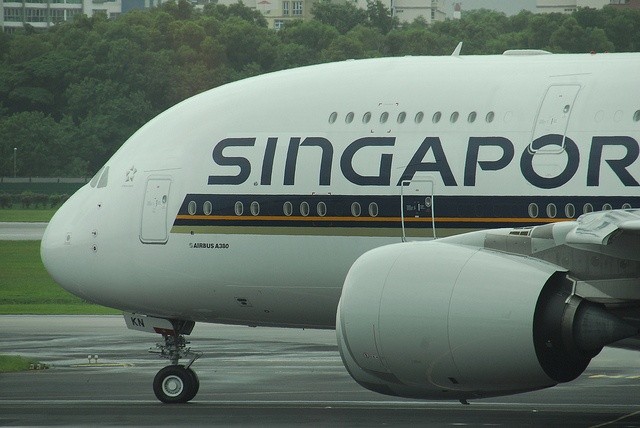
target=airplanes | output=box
[39,40,640,403]
